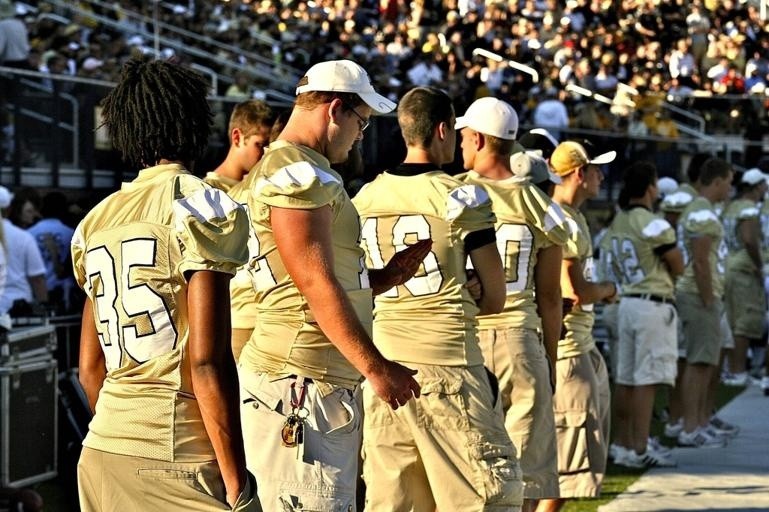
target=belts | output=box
[289,374,314,383]
[621,292,673,304]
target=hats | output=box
[509,150,549,184]
[658,191,695,213]
[657,176,680,199]
[352,45,370,55]
[563,40,576,47]
[0,186,15,210]
[294,58,399,114]
[741,167,765,186]
[446,10,457,21]
[421,42,433,54]
[549,136,617,178]
[527,38,542,50]
[519,127,560,161]
[560,16,571,25]
[453,96,520,142]
[15,4,267,102]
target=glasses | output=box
[343,101,371,131]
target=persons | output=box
[1,1,769,512]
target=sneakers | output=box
[607,435,679,471]
[706,415,740,436]
[677,423,728,448]
[719,368,769,397]
[653,409,684,438]
